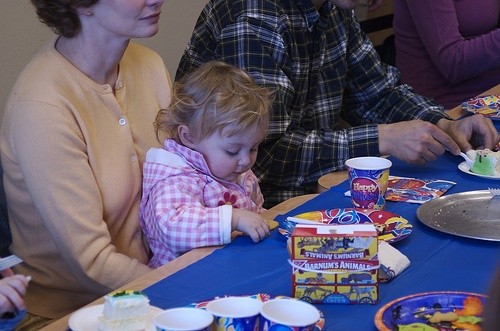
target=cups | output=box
[258,298,321,331]
[153,307,213,331]
[344,157,392,211]
[206,297,263,331]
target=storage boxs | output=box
[291,223,380,306]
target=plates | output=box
[278,208,413,244]
[460,95,500,120]
[373,291,489,331]
[69,304,166,331]
[458,160,500,180]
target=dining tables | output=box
[38,83,500,331]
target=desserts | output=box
[470,149,500,176]
[102,289,150,322]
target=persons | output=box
[392,0,500,111]
[0,0,178,315]
[0,271,32,317]
[140,58,271,268]
[175,0,500,209]
